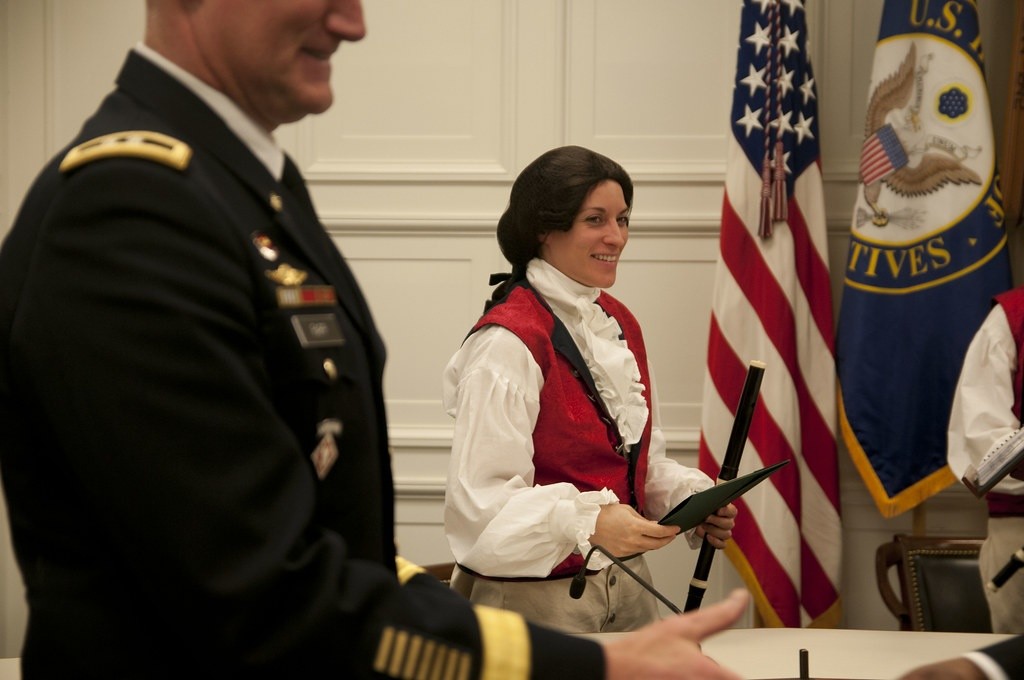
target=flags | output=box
[837,0,1012,518]
[697,1,842,631]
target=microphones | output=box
[569,545,683,617]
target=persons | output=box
[446,146,738,634]
[895,634,1024,680]
[0,1,751,680]
[946,284,1024,635]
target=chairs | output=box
[875,532,994,634]
